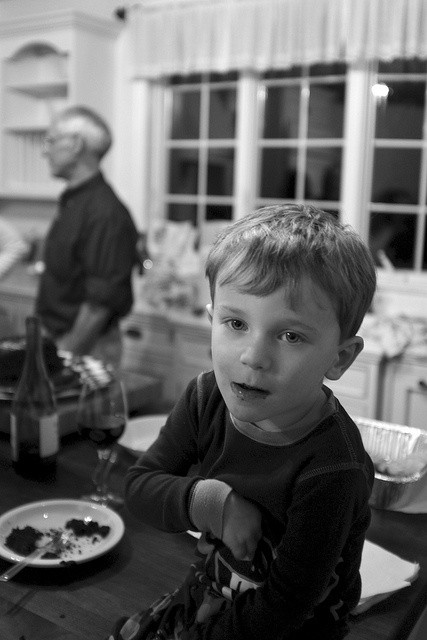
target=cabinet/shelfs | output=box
[321,354,379,423]
[384,361,427,518]
[1,11,121,199]
[119,317,209,408]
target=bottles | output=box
[8,314,61,488]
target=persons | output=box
[35,107,138,377]
[0,215,31,280]
[121,203,374,640]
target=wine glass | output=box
[74,375,129,511]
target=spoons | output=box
[0,531,72,583]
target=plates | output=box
[115,412,171,459]
[0,496,126,569]
